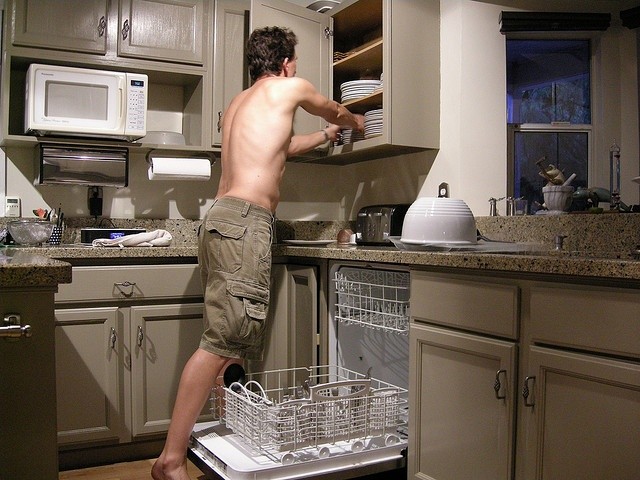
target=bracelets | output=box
[321,130,328,141]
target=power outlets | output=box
[87,186,103,216]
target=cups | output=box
[514,200,528,216]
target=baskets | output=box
[7,220,54,244]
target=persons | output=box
[151,26,365,480]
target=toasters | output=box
[355,203,412,246]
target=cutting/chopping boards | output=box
[333,51,356,62]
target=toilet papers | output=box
[148,156,210,182]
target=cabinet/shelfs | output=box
[2,0,207,77]
[53,263,246,471]
[247,0,438,165]
[406,271,640,479]
[204,3,247,151]
[251,264,321,404]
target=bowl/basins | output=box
[6,221,55,247]
[400,197,478,245]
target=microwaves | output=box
[24,63,149,141]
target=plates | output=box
[340,80,380,104]
[334,132,343,146]
[342,129,365,145]
[374,87,383,93]
[282,240,339,244]
[363,109,383,139]
